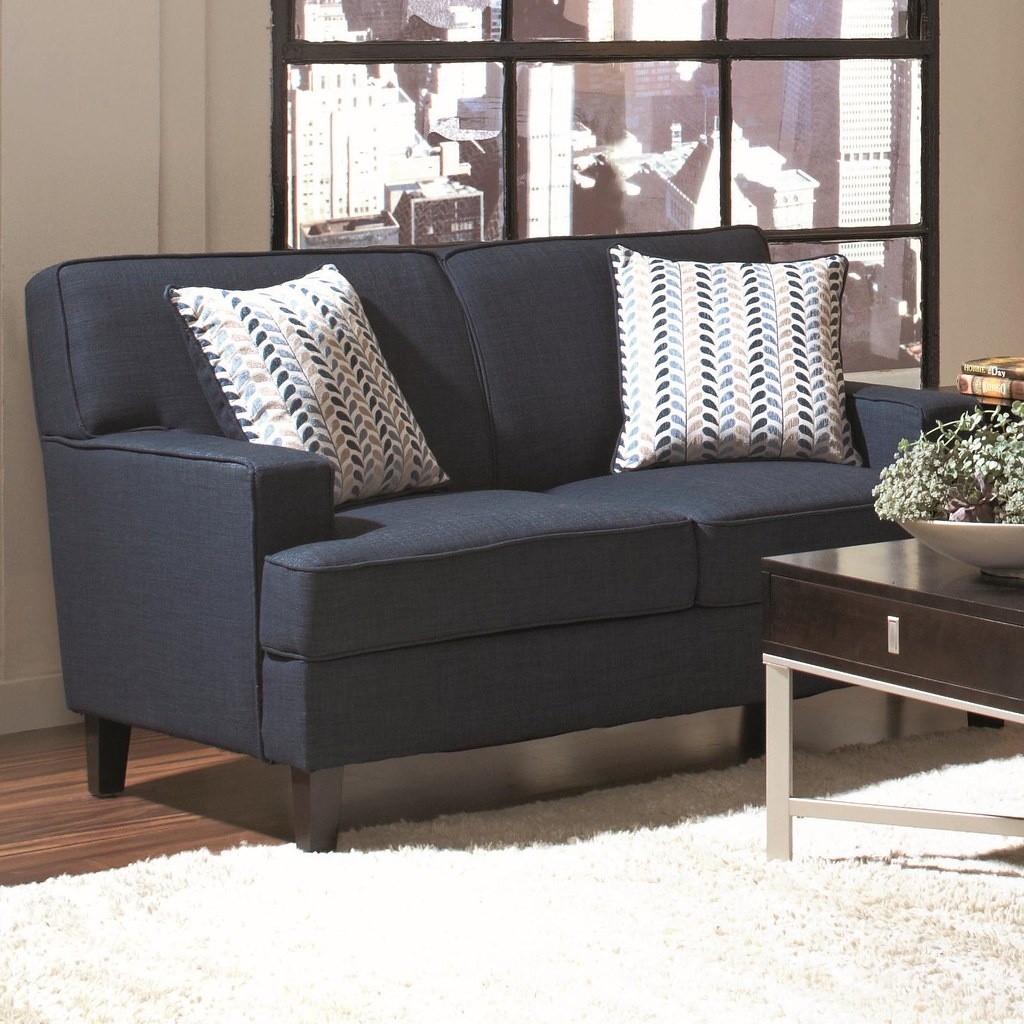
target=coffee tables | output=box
[753,537,1024,864]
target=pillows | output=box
[599,241,868,474]
[162,264,451,508]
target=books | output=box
[956,356,1024,401]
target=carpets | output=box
[1,756,1024,1024]
[1,711,1024,899]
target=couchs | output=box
[23,224,995,856]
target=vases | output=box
[892,518,1024,578]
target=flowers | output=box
[871,404,1024,521]
